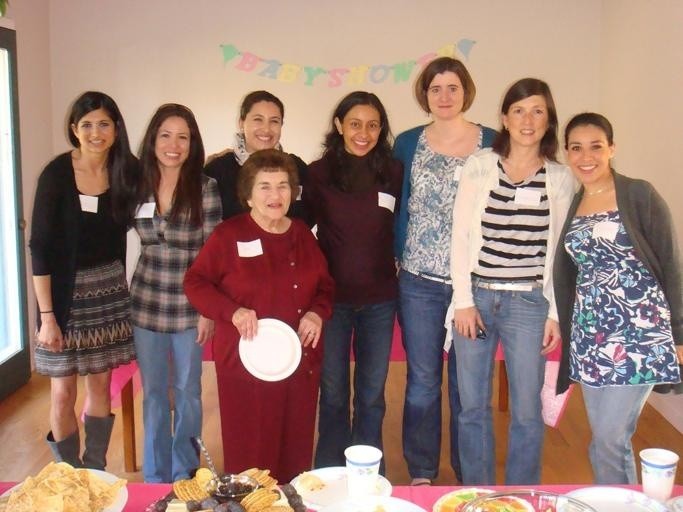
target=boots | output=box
[83,413,115,470]
[46,428,82,468]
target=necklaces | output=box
[583,180,612,198]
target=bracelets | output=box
[39,309,54,316]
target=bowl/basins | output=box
[205,474,259,501]
[457,490,594,512]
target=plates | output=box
[0,467,129,512]
[289,467,393,512]
[316,495,425,512]
[238,318,302,382]
[432,488,534,512]
[562,486,668,512]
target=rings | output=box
[309,330,316,336]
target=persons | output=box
[119,103,224,483]
[183,149,335,484]
[552,113,682,484]
[443,77,578,484]
[289,91,404,478]
[30,91,142,468]
[205,90,318,232]
[394,56,506,486]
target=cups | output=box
[343,444,384,476]
[639,448,679,501]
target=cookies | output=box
[172,468,293,512]
[0,461,128,512]
